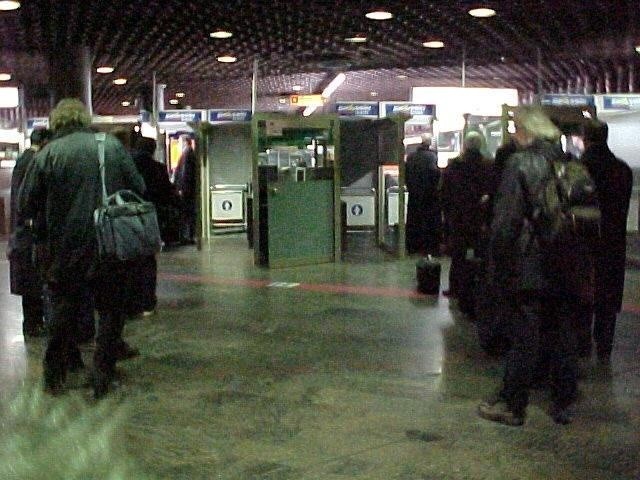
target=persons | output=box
[10,125,53,336]
[66,125,197,370]
[441,131,525,353]
[568,123,634,366]
[403,139,443,257]
[479,114,587,425]
[17,99,151,401]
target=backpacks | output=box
[520,145,602,254]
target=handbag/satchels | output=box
[93,189,162,263]
[416,254,441,295]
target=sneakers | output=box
[116,340,140,361]
[479,399,526,426]
